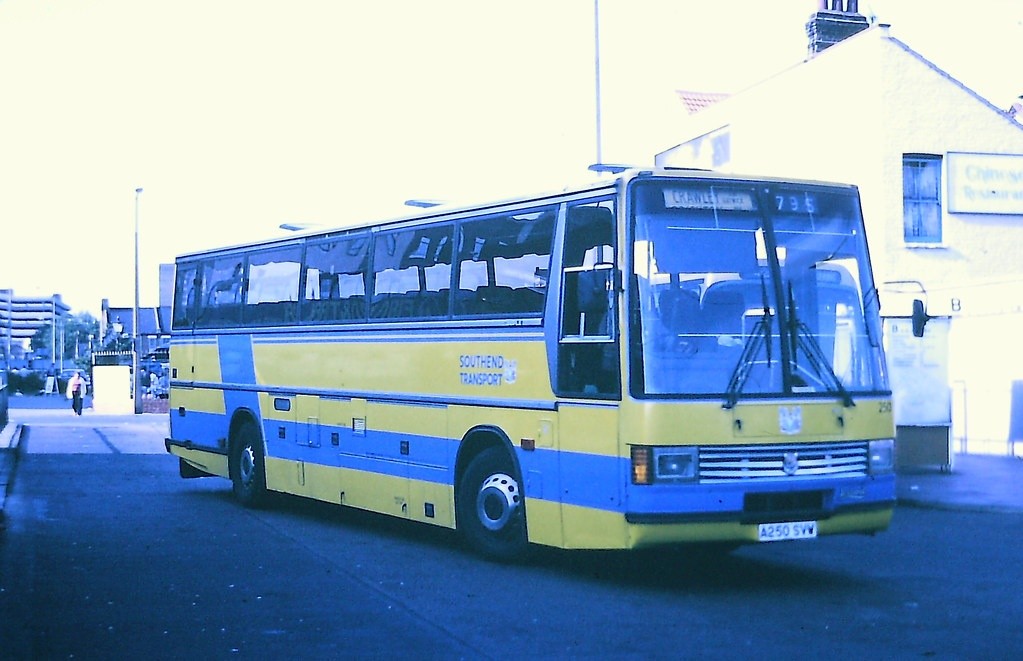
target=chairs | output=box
[196,280,725,332]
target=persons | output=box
[140,366,170,413]
[66,371,87,415]
[0,362,56,391]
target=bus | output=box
[165,162,932,565]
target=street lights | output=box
[131,186,146,414]
